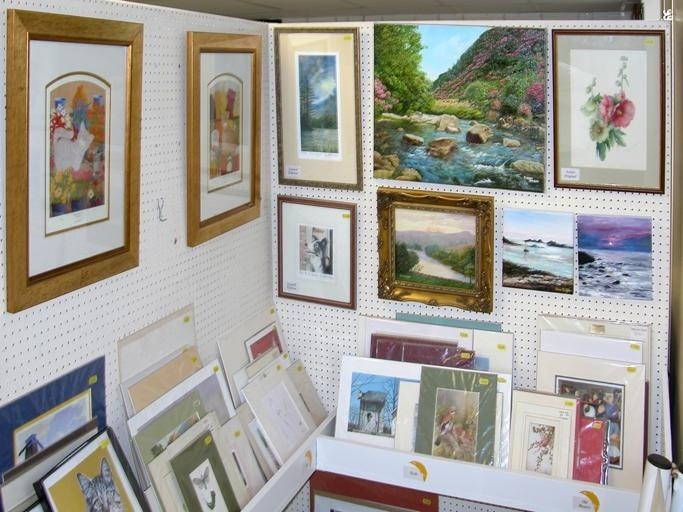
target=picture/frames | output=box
[377,188,492,316]
[187,31,261,247]
[274,26,363,193]
[552,29,666,195]
[5,9,144,313]
[277,194,356,310]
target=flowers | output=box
[581,56,635,163]
